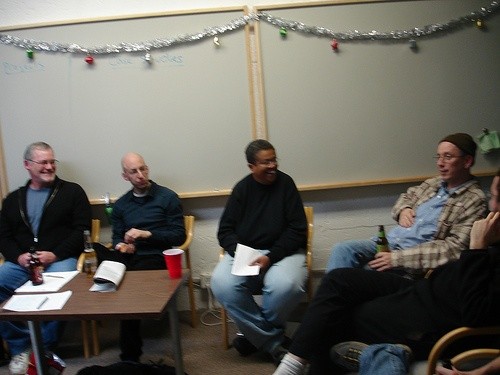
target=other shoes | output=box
[8,347,32,375]
[272,354,311,375]
[234,336,258,356]
[275,351,289,366]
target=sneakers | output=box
[329,341,412,372]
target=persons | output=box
[326,134,487,271]
[274,169,500,375]
[0,141,93,375]
[210,139,309,363]
[86,153,186,366]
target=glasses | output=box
[25,158,59,165]
[432,156,463,163]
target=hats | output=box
[439,133,477,158]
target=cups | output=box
[162,249,184,279]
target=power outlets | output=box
[200,273,211,288]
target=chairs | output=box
[330,268,500,375]
[180,216,197,329]
[0,219,100,359]
[220,207,314,350]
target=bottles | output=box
[376,224,389,254]
[104,192,113,224]
[30,247,43,285]
[83,230,98,282]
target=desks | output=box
[0,269,191,375]
